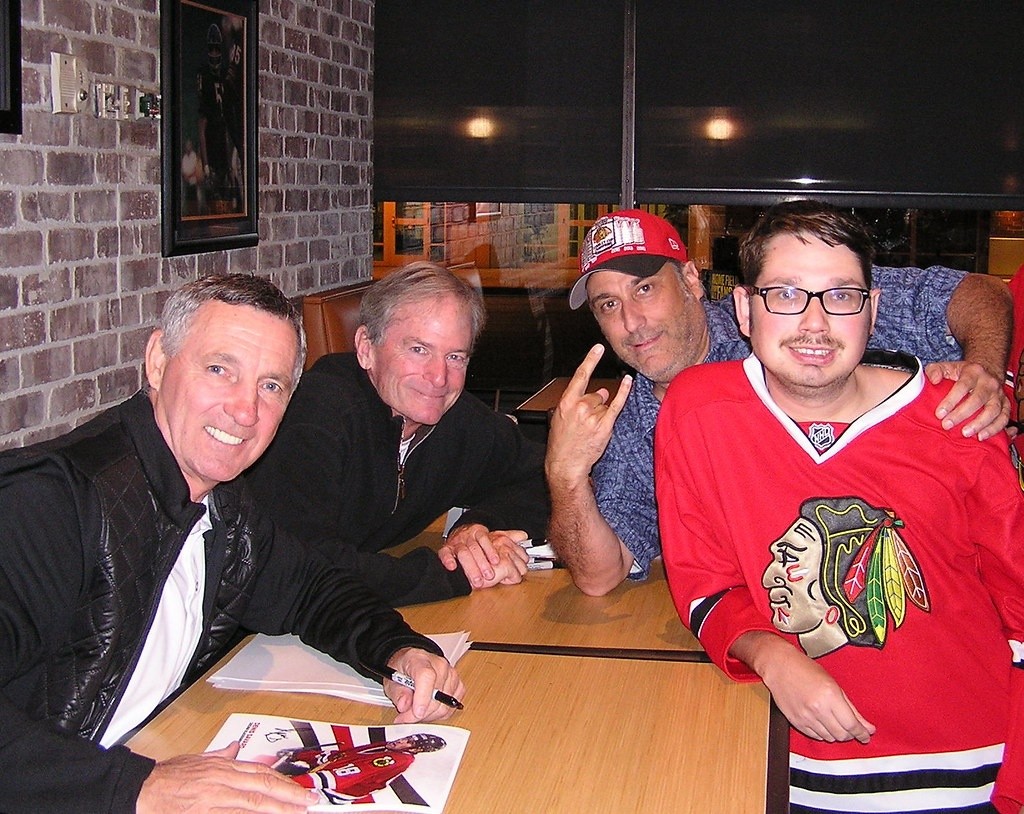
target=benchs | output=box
[303,280,376,372]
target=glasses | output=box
[733,283,871,315]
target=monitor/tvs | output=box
[373,0,1024,211]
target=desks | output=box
[125,635,771,814]
[377,377,712,663]
[513,378,623,425]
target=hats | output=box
[569,209,687,310]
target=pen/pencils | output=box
[527,557,543,564]
[358,654,464,710]
[526,561,560,571]
[517,538,548,548]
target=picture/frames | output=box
[160,0,260,258]
[475,203,503,218]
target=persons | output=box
[180,15,244,217]
[256,732,444,806]
[0,273,465,814]
[546,197,1024,814]
[223,261,550,653]
